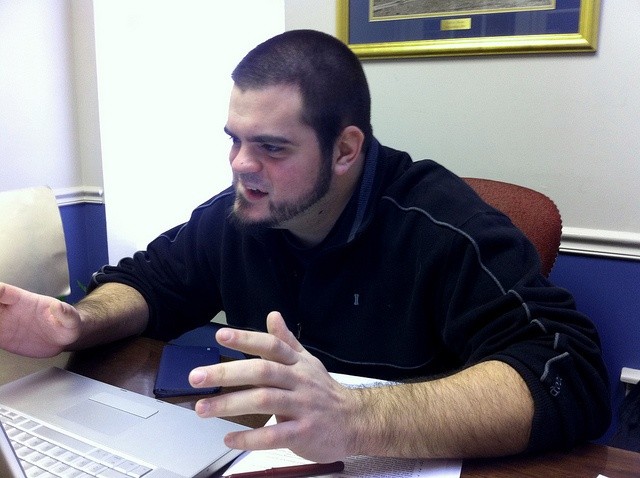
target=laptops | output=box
[1,367,255,478]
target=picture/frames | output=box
[335,0,600,61]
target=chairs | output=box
[461,177,562,280]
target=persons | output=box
[0,30,611,463]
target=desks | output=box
[0,297,639,478]
